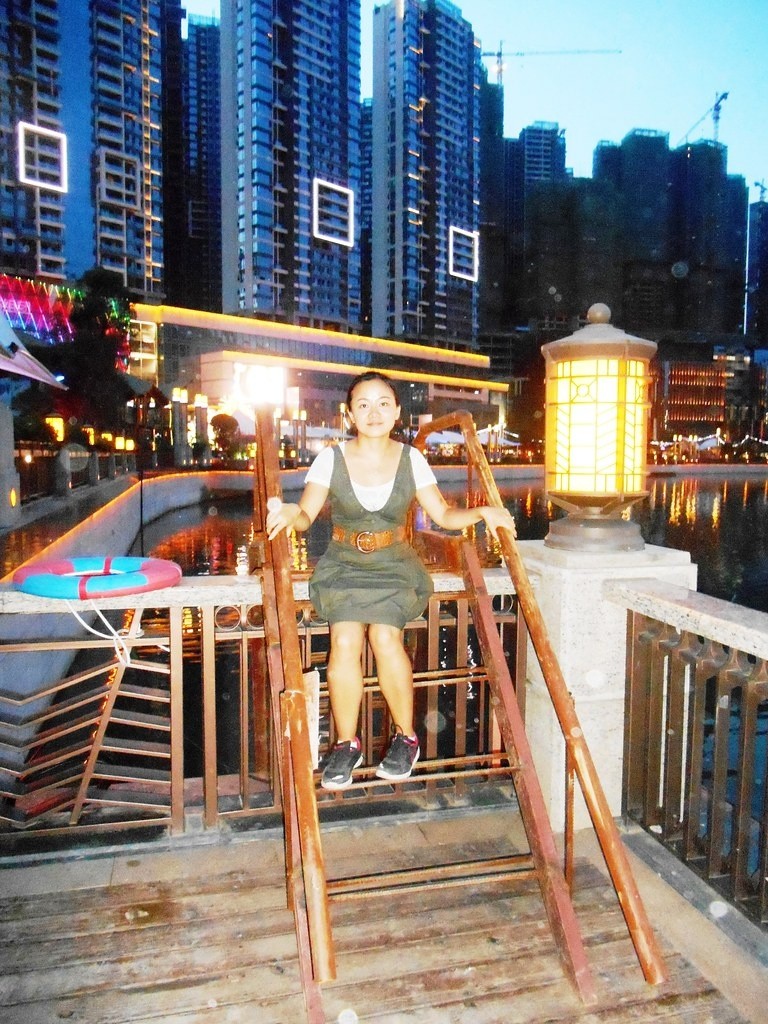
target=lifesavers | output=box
[12,556,182,600]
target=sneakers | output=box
[374,723,420,780]
[320,737,363,789]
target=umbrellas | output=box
[232,409,523,463]
[0,328,169,436]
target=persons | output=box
[266,371,517,789]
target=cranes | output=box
[480,39,623,136]
[754,178,768,203]
[674,90,729,148]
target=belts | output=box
[332,524,406,554]
